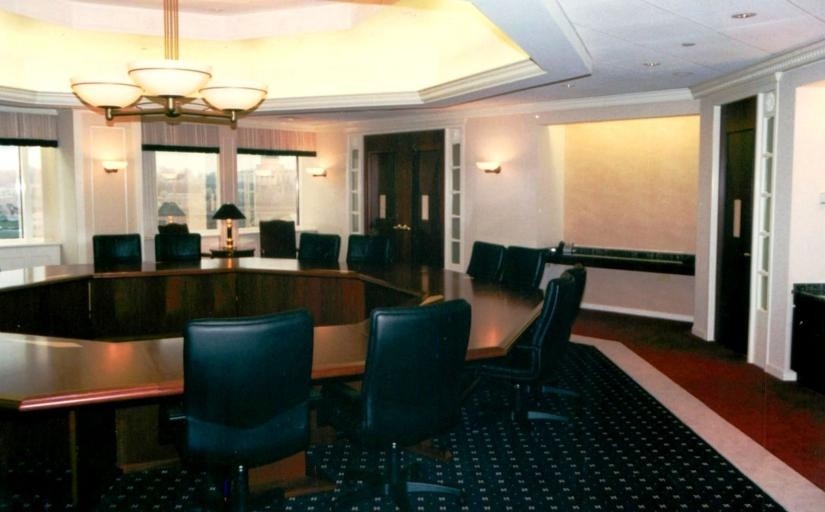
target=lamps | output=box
[211,203,246,251]
[475,161,501,173]
[69,0,267,124]
[306,167,327,177]
[103,160,128,173]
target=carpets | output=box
[0,335,825,512]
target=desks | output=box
[0,255,548,512]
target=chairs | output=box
[184,304,332,511]
[89,217,546,289]
[464,266,586,467]
[340,299,477,509]
